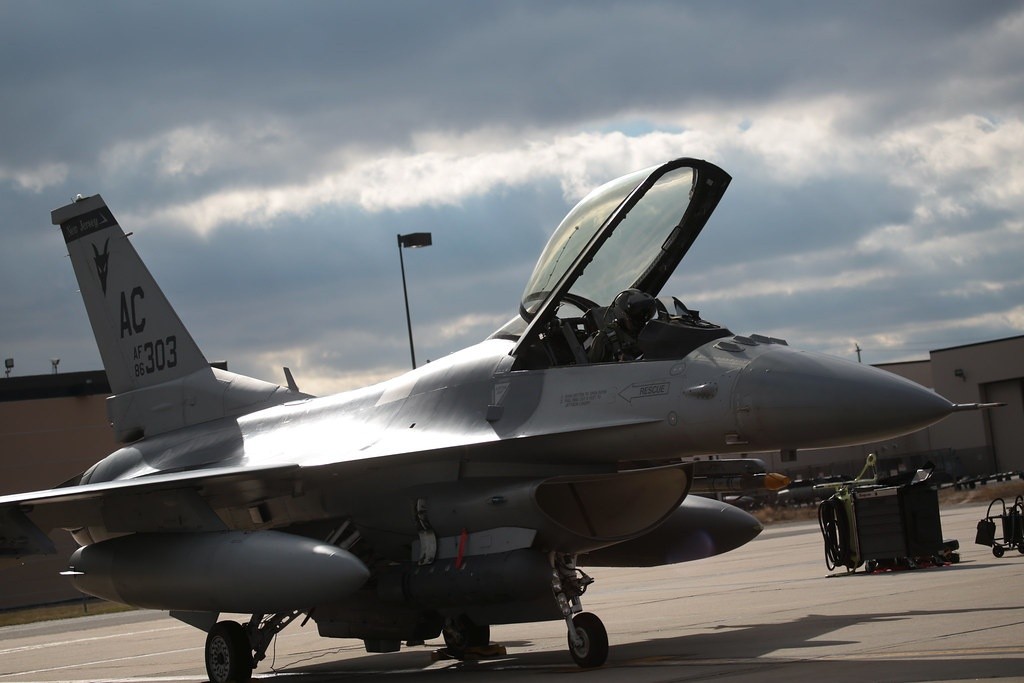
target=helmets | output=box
[615,290,656,332]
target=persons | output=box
[585,288,657,362]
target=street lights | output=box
[398,233,432,369]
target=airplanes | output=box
[0,157,1007,683]
